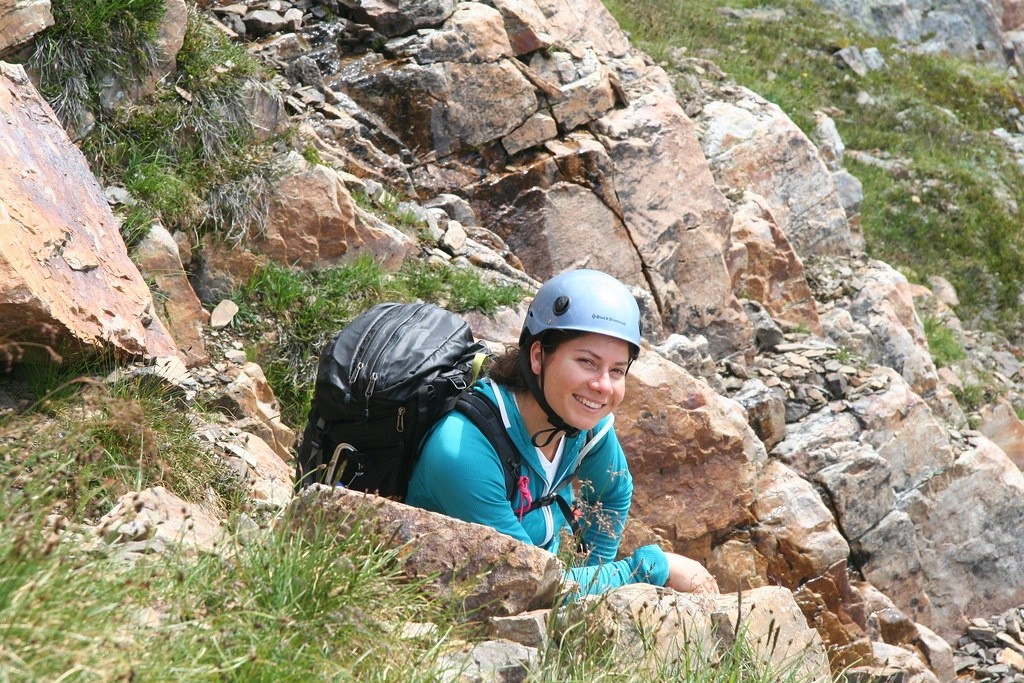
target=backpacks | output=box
[297,303,593,504]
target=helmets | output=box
[519,269,641,362]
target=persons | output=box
[406,269,720,606]
